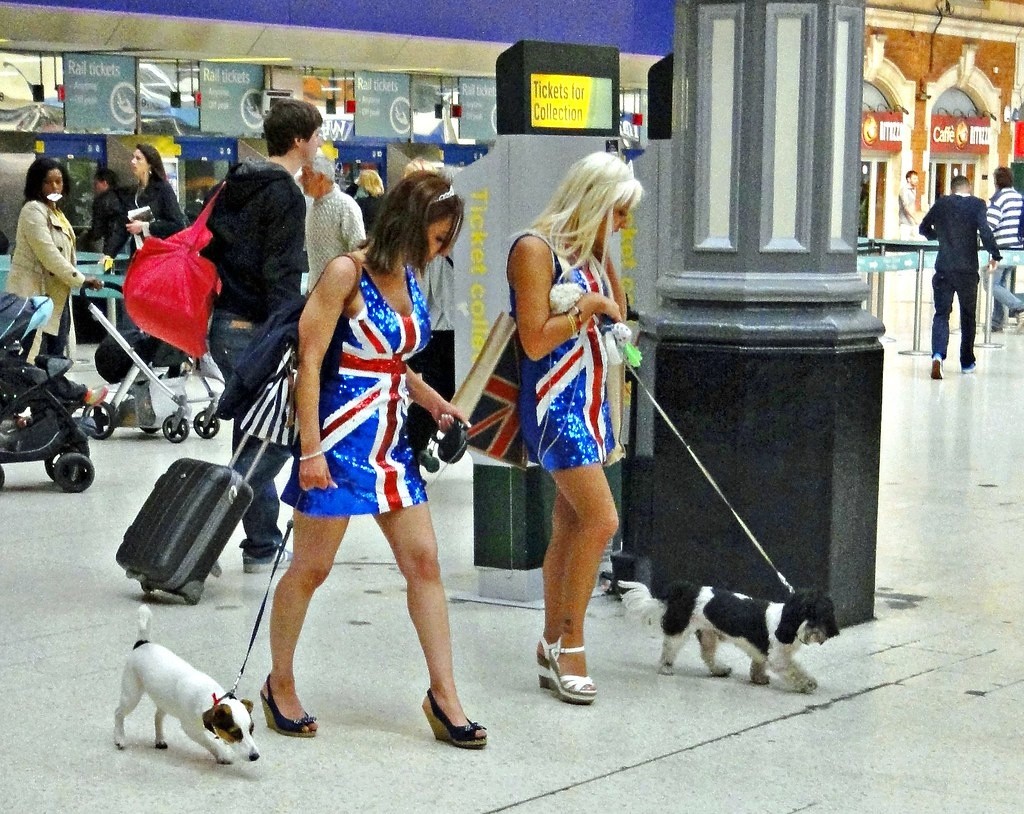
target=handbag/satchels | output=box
[95,331,151,384]
[180,213,190,230]
[122,180,222,357]
[590,252,627,468]
[237,253,362,446]
[449,310,528,471]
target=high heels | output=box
[550,634,597,703]
[260,673,316,737]
[536,636,556,688]
[422,688,488,746]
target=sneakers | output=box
[244,548,294,572]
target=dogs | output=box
[114,605,262,764]
[616,581,840,694]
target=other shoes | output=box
[85,387,107,405]
[1015,311,1024,334]
[982,326,1003,332]
[931,353,944,379]
[961,362,976,373]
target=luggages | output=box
[116,431,271,604]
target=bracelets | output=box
[567,306,582,339]
[300,452,322,461]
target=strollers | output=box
[0,289,98,494]
[78,280,228,445]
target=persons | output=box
[507,150,646,705]
[403,157,462,459]
[977,167,1024,335]
[76,168,132,252]
[261,170,488,750]
[0,347,109,407]
[898,171,922,242]
[100,144,187,330]
[6,157,103,368]
[920,175,1002,379]
[345,162,384,231]
[298,157,366,294]
[201,99,323,577]
